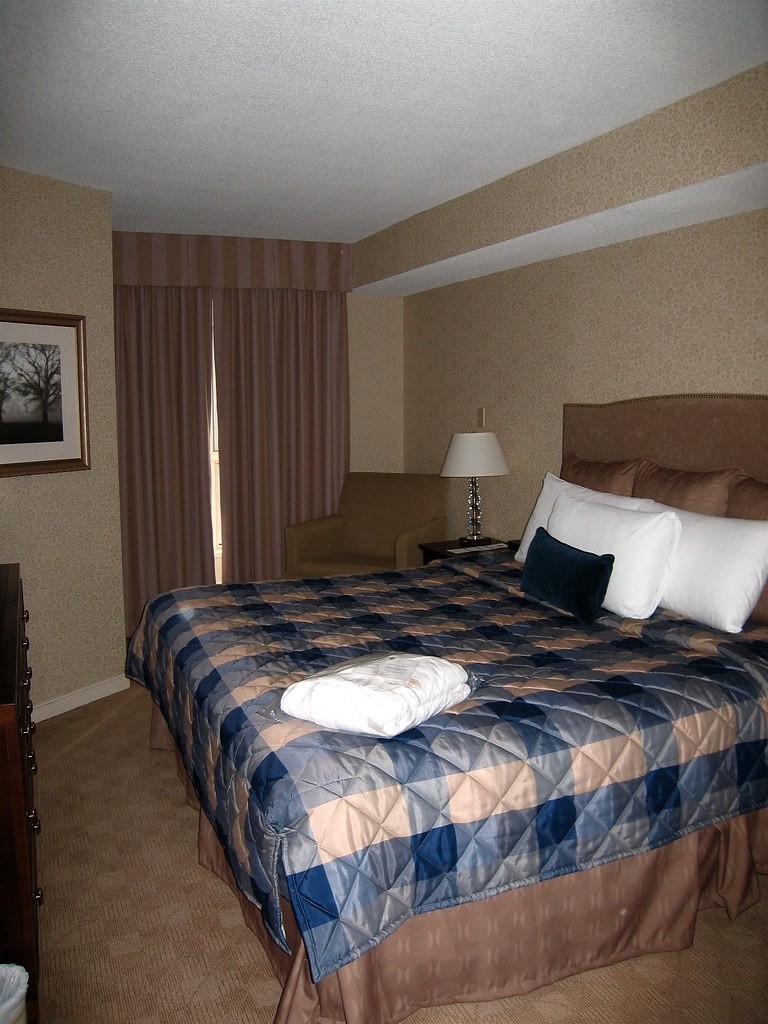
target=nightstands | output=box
[417,536,506,565]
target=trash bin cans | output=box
[0,963,27,1024]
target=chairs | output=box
[285,471,451,578]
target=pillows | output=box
[514,472,655,563]
[640,499,768,635]
[727,472,768,520]
[631,457,745,516]
[520,527,615,630]
[560,452,642,496]
[549,493,683,620]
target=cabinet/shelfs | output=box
[0,562,44,1024]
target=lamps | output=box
[438,432,510,545]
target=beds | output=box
[122,393,768,1024]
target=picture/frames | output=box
[0,307,92,478]
